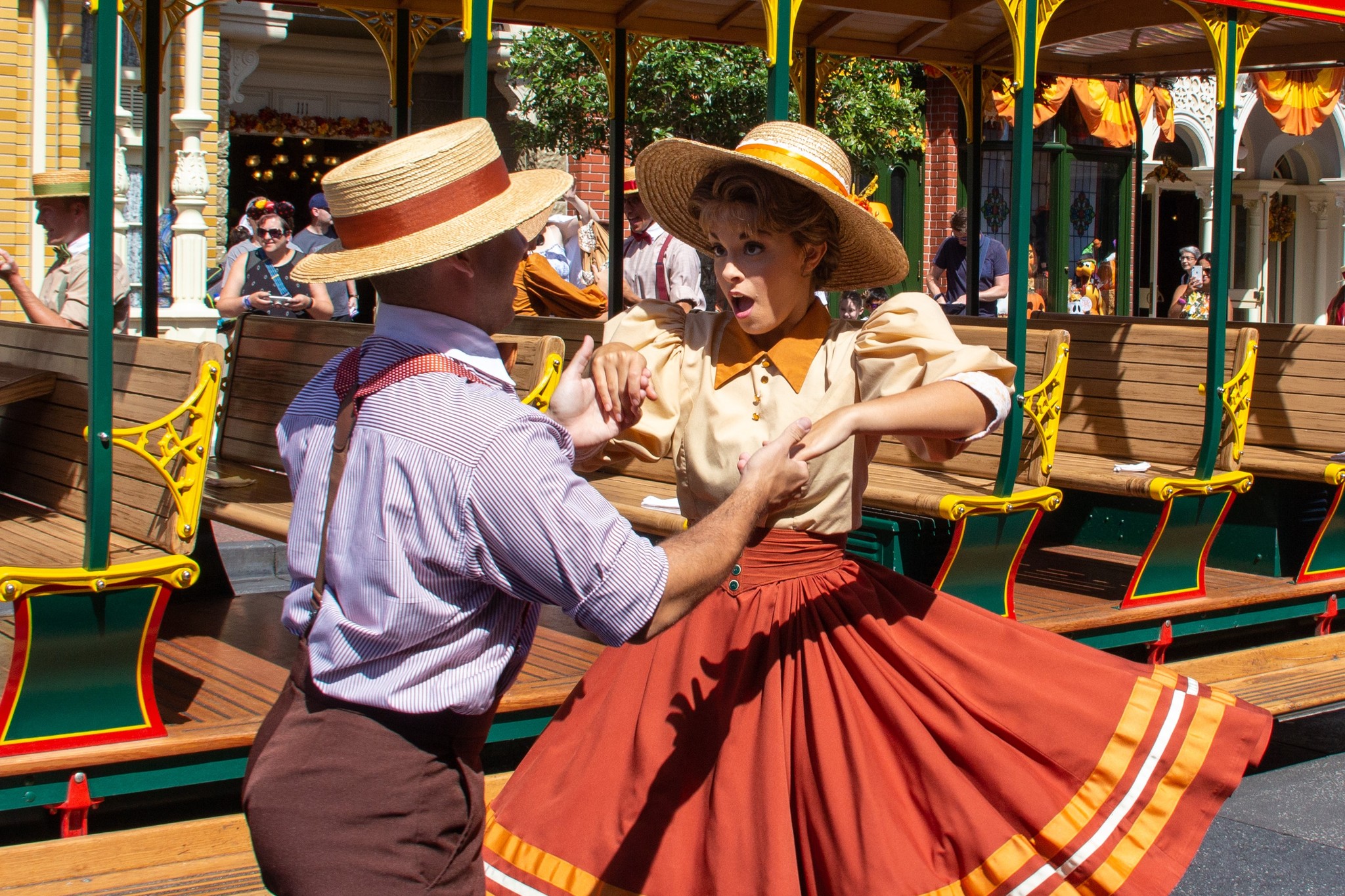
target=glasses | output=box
[1179,256,1196,260]
[868,303,878,309]
[256,227,290,237]
[952,234,968,241]
[1202,268,1211,275]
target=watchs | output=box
[349,295,359,299]
[306,298,313,309]
[1183,295,1188,298]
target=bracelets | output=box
[241,295,253,311]
[1178,298,1186,304]
[934,293,944,301]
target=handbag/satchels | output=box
[939,304,967,316]
[577,206,609,287]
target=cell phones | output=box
[1191,265,1204,287]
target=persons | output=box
[860,287,889,321]
[511,166,707,320]
[926,207,1009,317]
[593,124,1280,896]
[242,117,810,896]
[1168,253,1234,321]
[839,290,864,319]
[814,290,831,317]
[1326,266,1345,326]
[291,193,359,322]
[0,170,131,334]
[221,226,251,270]
[215,201,334,320]
[239,215,254,235]
[1179,246,1201,285]
[220,197,304,291]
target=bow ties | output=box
[52,244,73,265]
[632,232,652,245]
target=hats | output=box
[309,193,329,208]
[634,121,909,292]
[603,164,638,195]
[12,169,90,200]
[289,118,574,284]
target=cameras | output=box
[269,296,292,309]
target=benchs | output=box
[0,312,1345,756]
